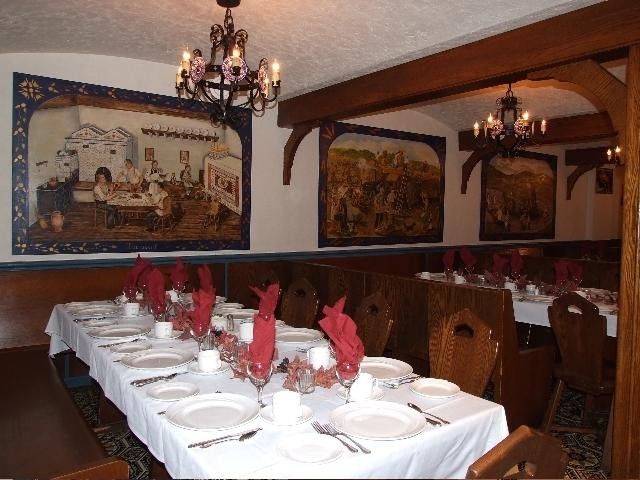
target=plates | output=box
[363,356,462,399]
[417,271,471,286]
[586,287,622,305]
[181,291,323,343]
[67,302,194,368]
[146,383,263,433]
[188,360,231,374]
[331,402,427,438]
[111,189,153,203]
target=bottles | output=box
[49,177,58,190]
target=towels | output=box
[118,253,217,339]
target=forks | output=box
[310,420,373,458]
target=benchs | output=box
[0,339,136,479]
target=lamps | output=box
[472,79,549,160]
[605,142,624,169]
[174,0,284,133]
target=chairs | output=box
[227,247,619,480]
[91,167,224,234]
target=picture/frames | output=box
[478,148,559,242]
[9,69,255,257]
[594,167,614,195]
[316,115,450,250]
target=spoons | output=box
[187,427,264,452]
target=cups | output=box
[297,368,318,395]
[306,345,330,370]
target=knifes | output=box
[408,403,450,428]
[130,370,179,388]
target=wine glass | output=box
[443,265,587,300]
[330,336,360,407]
[194,326,280,407]
[121,279,213,351]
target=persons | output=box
[146,160,164,191]
[329,149,531,234]
[145,183,169,231]
[116,158,144,191]
[180,165,193,197]
[94,172,123,228]
